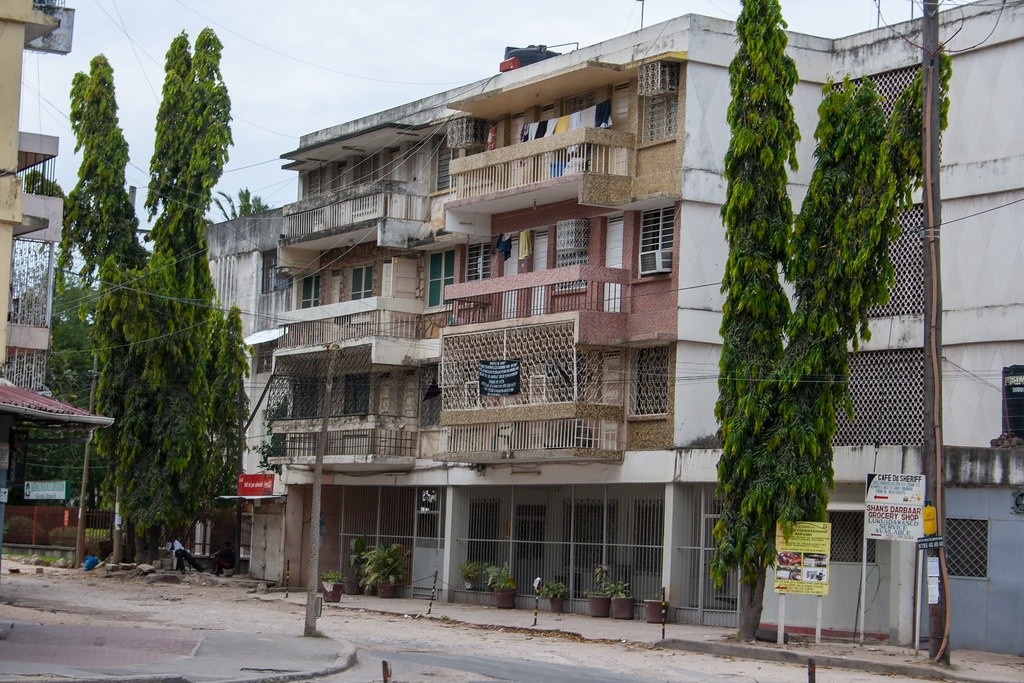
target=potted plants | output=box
[539,581,569,614]
[609,577,634,620]
[643,600,669,624]
[586,565,611,617]
[321,536,405,602]
[459,560,518,610]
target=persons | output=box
[209,542,235,576]
[166,535,207,574]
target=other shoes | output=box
[199,565,208,573]
[182,568,186,574]
